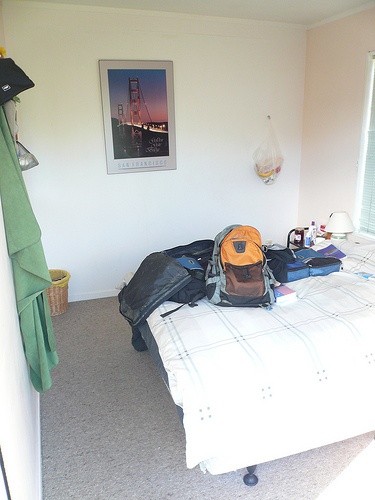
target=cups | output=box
[313,238,325,245]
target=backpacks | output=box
[165,254,205,304]
[205,224,280,307]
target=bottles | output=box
[294,220,323,247]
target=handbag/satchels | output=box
[253,119,284,185]
[266,246,342,283]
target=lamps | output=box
[325,211,355,240]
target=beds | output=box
[119,233,375,486]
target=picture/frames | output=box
[99,60,178,174]
